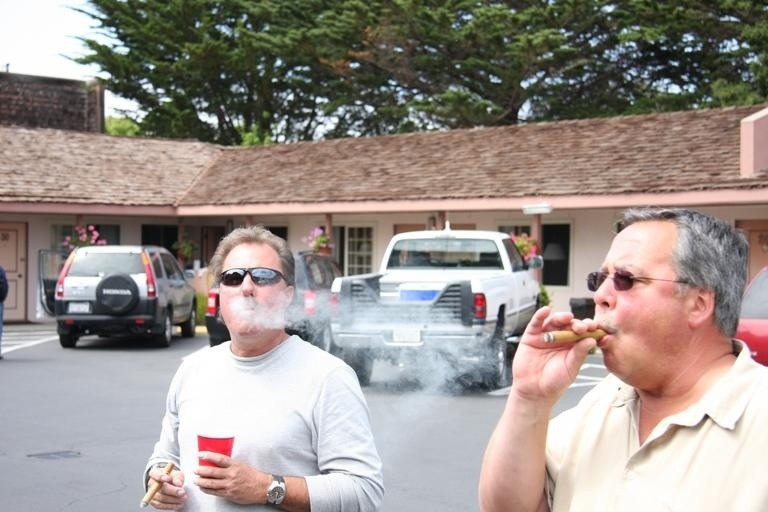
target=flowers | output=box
[300,227,336,253]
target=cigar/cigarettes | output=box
[139,462,176,510]
[542,327,608,345]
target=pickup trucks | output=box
[329,220,545,392]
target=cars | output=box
[729,265,768,366]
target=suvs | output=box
[206,250,353,357]
[34,242,200,348]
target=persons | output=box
[0,264,9,359]
[476,206,768,512]
[137,223,386,511]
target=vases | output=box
[319,247,331,255]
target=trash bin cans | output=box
[569,298,596,320]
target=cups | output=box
[197,430,237,480]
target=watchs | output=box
[266,473,287,507]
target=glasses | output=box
[584,267,676,292]
[217,266,292,286]
